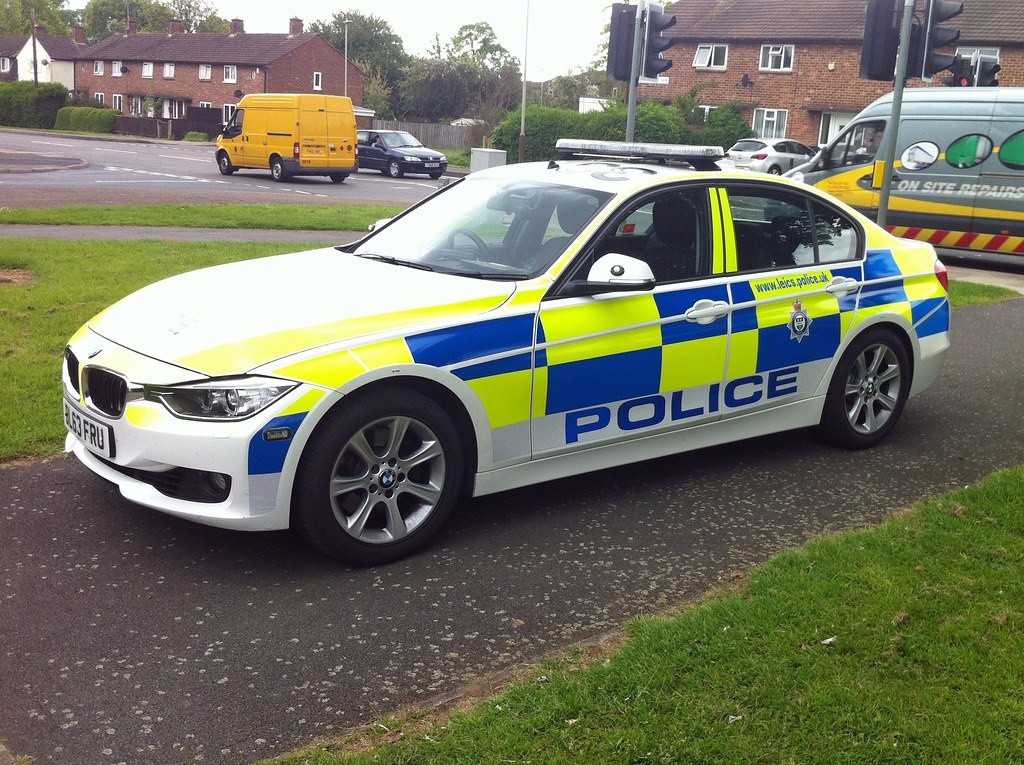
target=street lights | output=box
[343,20,354,97]
[30,7,39,88]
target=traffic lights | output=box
[915,1,964,85]
[939,52,974,87]
[639,3,679,82]
[974,56,1002,87]
[857,0,897,81]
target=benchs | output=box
[733,222,842,269]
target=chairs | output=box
[531,196,603,280]
[649,197,699,280]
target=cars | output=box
[59,137,951,572]
[726,136,817,179]
[357,129,448,179]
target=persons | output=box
[371,135,381,147]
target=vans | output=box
[778,85,1024,271]
[214,93,360,184]
